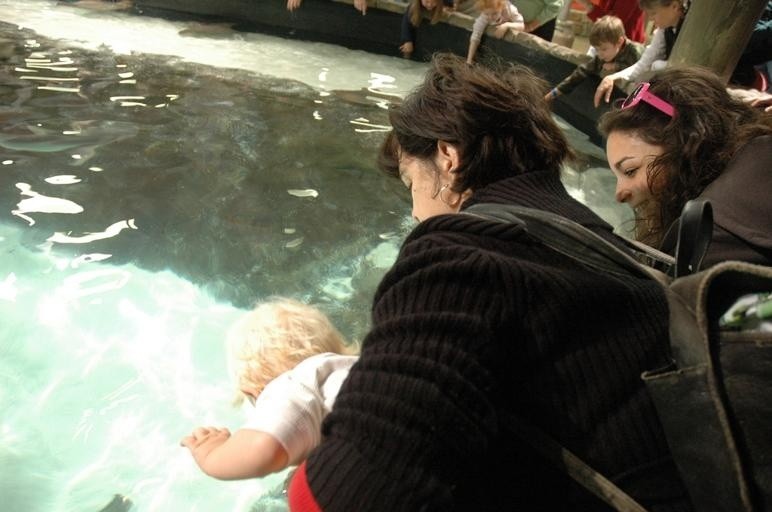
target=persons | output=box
[401,0,460,54]
[465,0,529,69]
[272,54,742,509]
[581,1,648,64]
[536,17,642,108]
[170,291,369,482]
[286,0,308,14]
[353,0,369,16]
[589,0,770,107]
[510,1,567,37]
[594,70,770,254]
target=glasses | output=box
[618,79,677,121]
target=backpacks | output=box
[442,201,772,512]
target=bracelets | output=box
[552,89,560,99]
[528,23,534,33]
